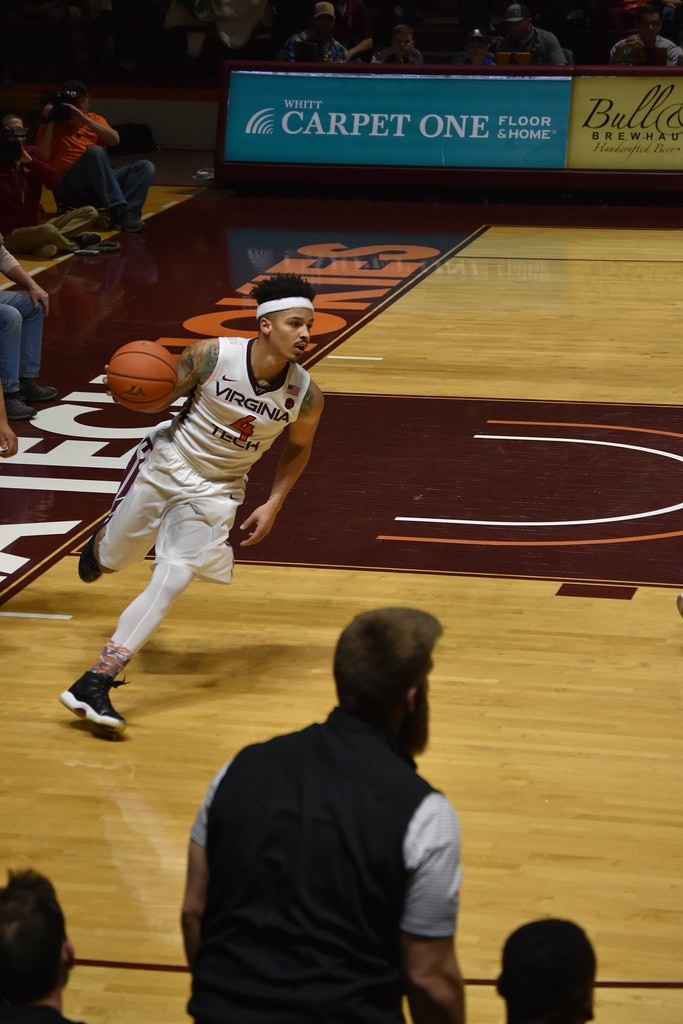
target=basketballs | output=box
[107,340,178,411]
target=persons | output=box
[59,273,324,734]
[370,24,424,63]
[0,232,58,421]
[451,29,496,65]
[497,919,595,1024]
[488,4,568,65]
[283,2,373,63]
[0,114,102,258]
[0,868,88,1024]
[35,80,156,233]
[0,379,18,458]
[182,607,466,1024]
[608,6,683,66]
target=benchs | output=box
[0,13,467,75]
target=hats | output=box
[463,29,490,46]
[313,2,335,17]
[61,80,88,101]
[502,4,531,21]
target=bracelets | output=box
[41,115,49,124]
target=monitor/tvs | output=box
[496,52,532,64]
[633,46,668,66]
[294,43,320,63]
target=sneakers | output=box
[99,214,114,228]
[79,526,107,583]
[116,219,146,233]
[5,396,37,419]
[62,670,132,734]
[18,384,57,400]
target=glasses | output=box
[641,21,660,28]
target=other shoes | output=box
[34,244,57,258]
[72,233,102,250]
